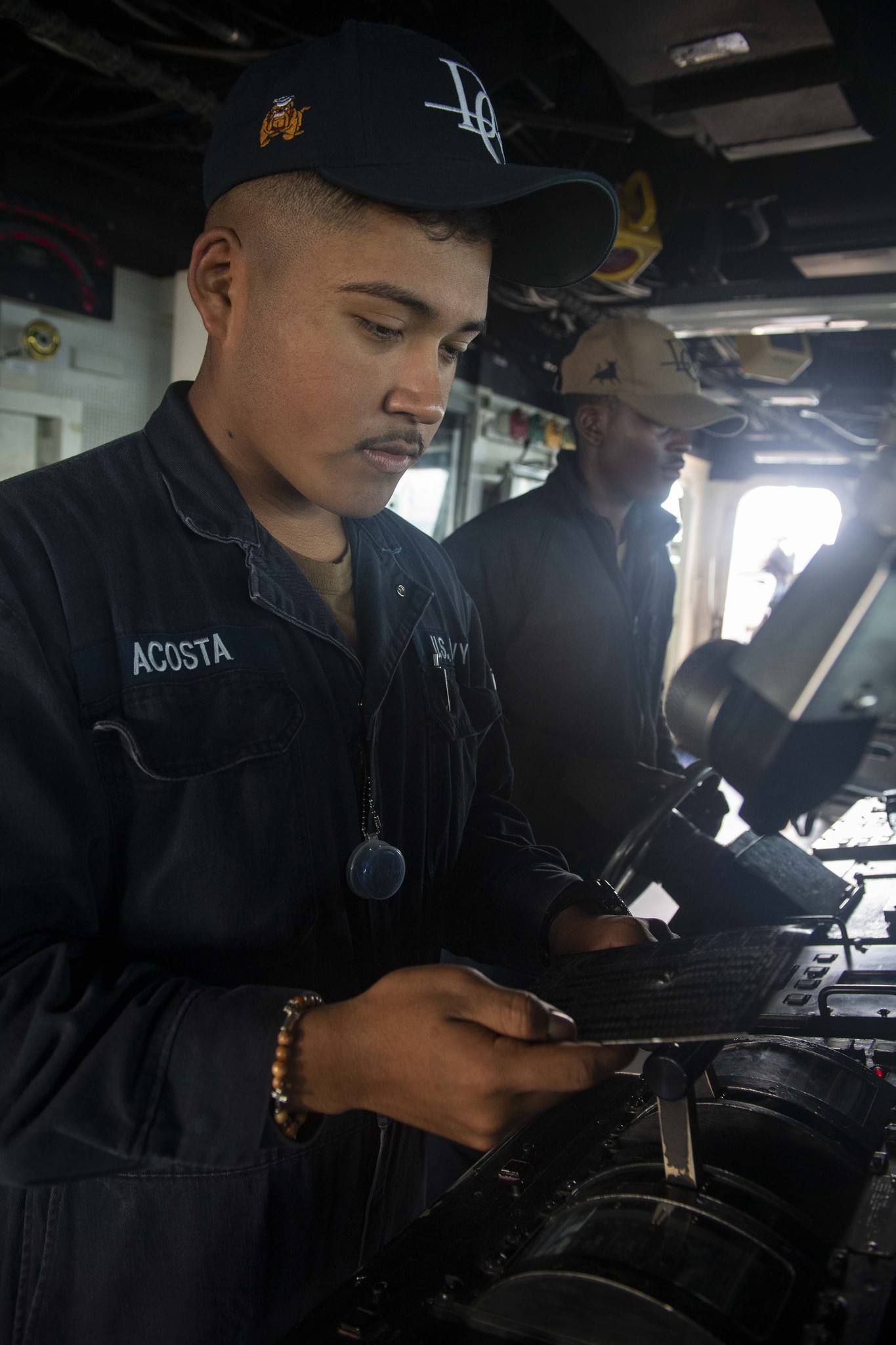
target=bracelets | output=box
[270,992,326,1143]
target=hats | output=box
[558,313,750,438]
[191,43,624,292]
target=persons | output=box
[0,69,685,1345]
[439,314,696,886]
